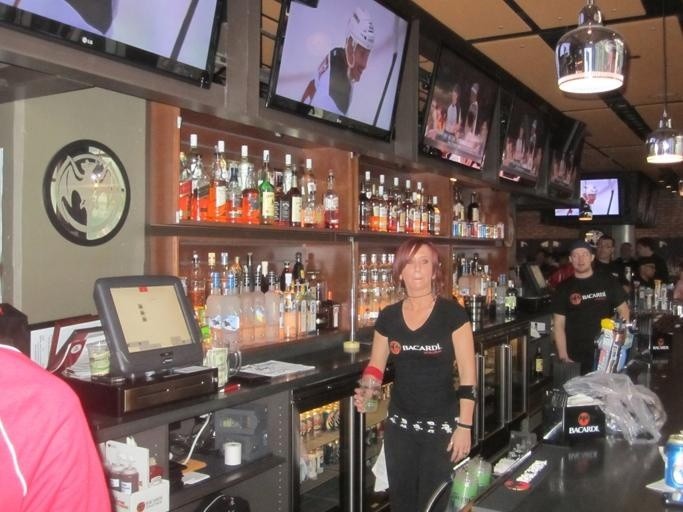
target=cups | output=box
[86,341,109,374]
[357,377,380,412]
[200,338,243,387]
[445,458,492,511]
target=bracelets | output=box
[456,421,472,430]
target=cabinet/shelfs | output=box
[69,374,289,512]
[262,305,558,510]
[148,102,356,361]
[352,151,450,321]
[451,177,511,301]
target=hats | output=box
[569,240,594,256]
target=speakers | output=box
[556,118,587,152]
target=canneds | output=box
[299,400,340,482]
[664,434,683,507]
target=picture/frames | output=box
[38,137,131,248]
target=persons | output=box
[518,236,683,302]
[566,184,597,215]
[0,341,114,511]
[298,6,376,118]
[526,120,537,169]
[441,84,461,145]
[552,240,630,374]
[463,83,477,138]
[352,237,478,512]
[513,122,525,164]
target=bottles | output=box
[187,248,340,348]
[534,345,544,378]
[451,187,483,223]
[357,253,403,325]
[95,454,161,493]
[177,133,340,230]
[450,252,519,317]
[358,167,442,235]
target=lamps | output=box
[552,1,625,96]
[643,2,682,165]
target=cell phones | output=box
[90,374,126,384]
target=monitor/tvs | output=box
[0,0,225,89]
[521,261,549,294]
[419,44,498,172]
[552,176,624,219]
[548,142,578,193]
[94,273,205,376]
[265,0,412,141]
[499,94,550,183]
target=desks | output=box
[460,429,683,510]
